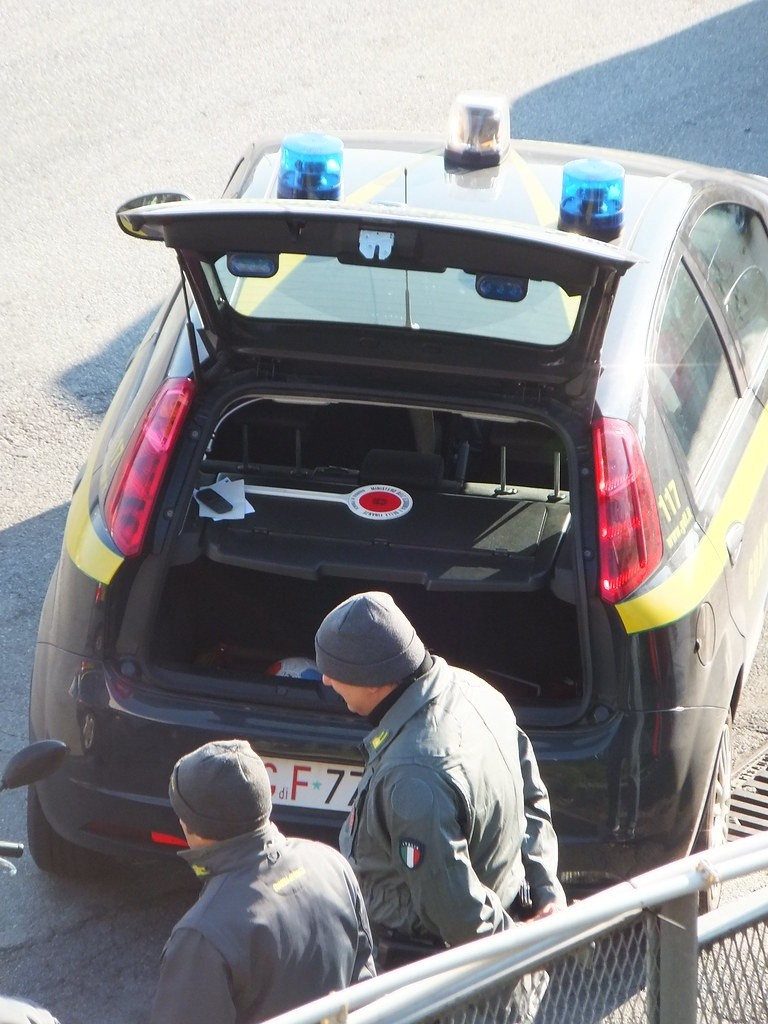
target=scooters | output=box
[0,739,72,1024]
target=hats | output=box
[314,590,425,688]
[169,739,273,840]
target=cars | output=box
[29,89,768,915]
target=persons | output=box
[141,738,376,1024]
[313,592,567,1023]
